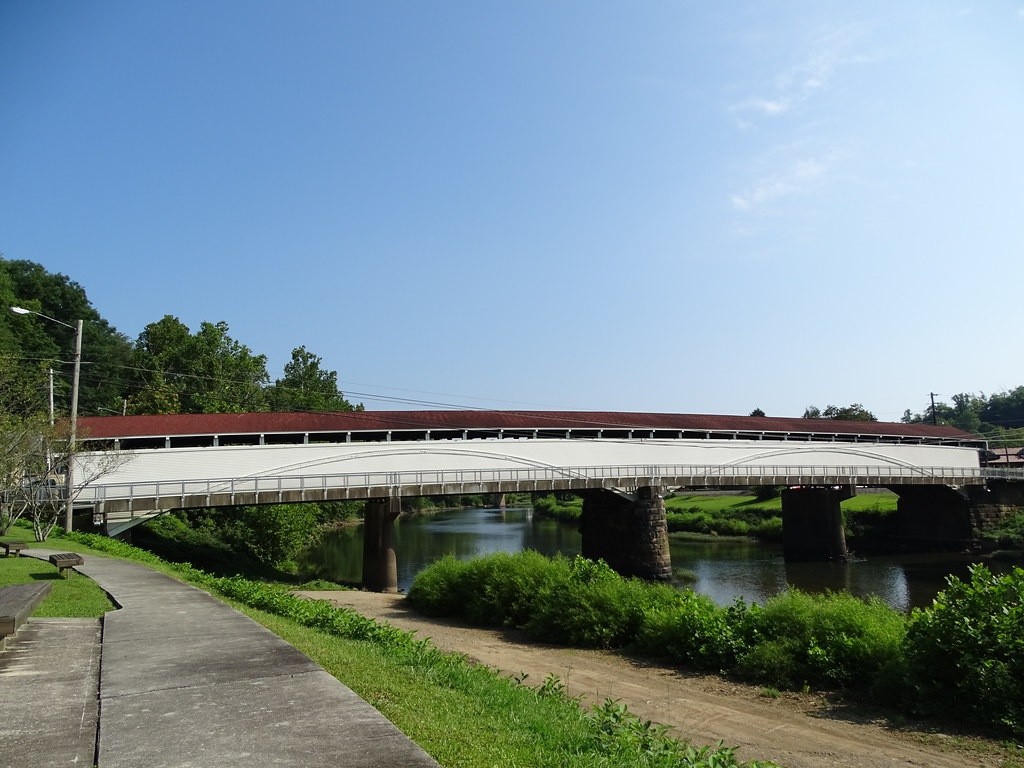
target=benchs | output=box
[49,552,85,580]
[0,582,53,650]
[0,540,30,559]
